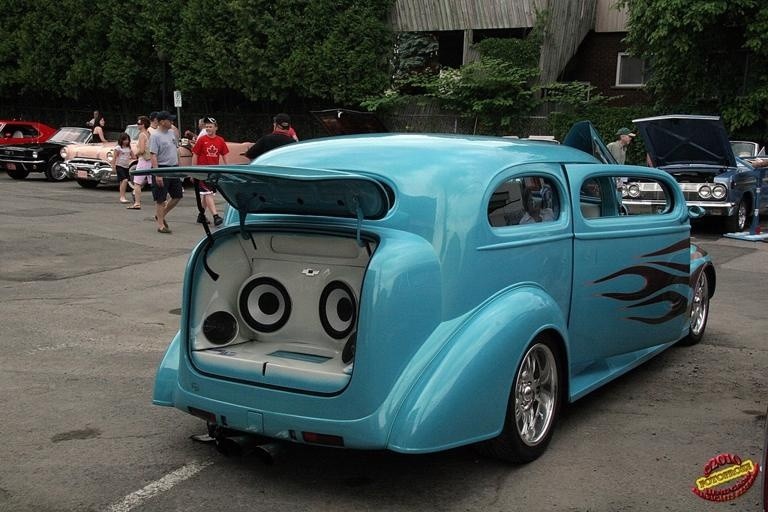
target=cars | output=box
[60,124,257,191]
[0,128,94,182]
[128,131,717,465]
[0,121,59,145]
[619,113,768,231]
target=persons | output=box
[86,109,180,235]
[190,117,228,224]
[195,118,208,144]
[517,185,557,225]
[606,127,637,164]
[246,112,295,166]
[288,128,299,144]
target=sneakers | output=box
[120,191,224,233]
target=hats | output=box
[616,127,636,136]
[157,111,177,122]
[204,117,218,127]
[273,113,291,131]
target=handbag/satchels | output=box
[143,140,151,161]
